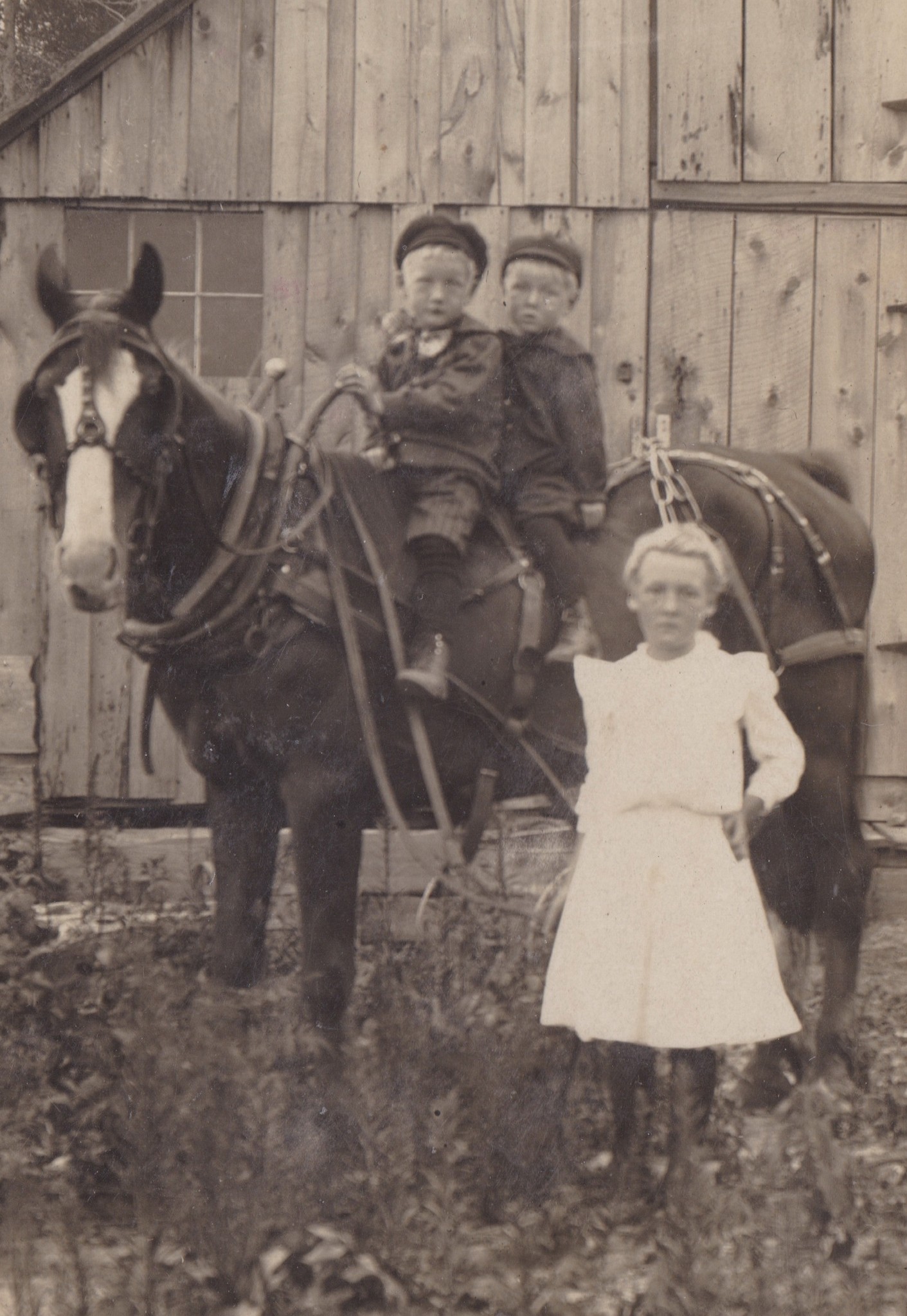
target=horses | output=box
[10,235,885,1125]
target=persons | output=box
[330,211,501,699]
[538,524,805,1229]
[493,236,608,666]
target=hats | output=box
[395,216,489,280]
[501,235,583,289]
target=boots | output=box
[394,633,448,700]
[583,1041,658,1225]
[544,597,601,666]
[662,1049,716,1227]
